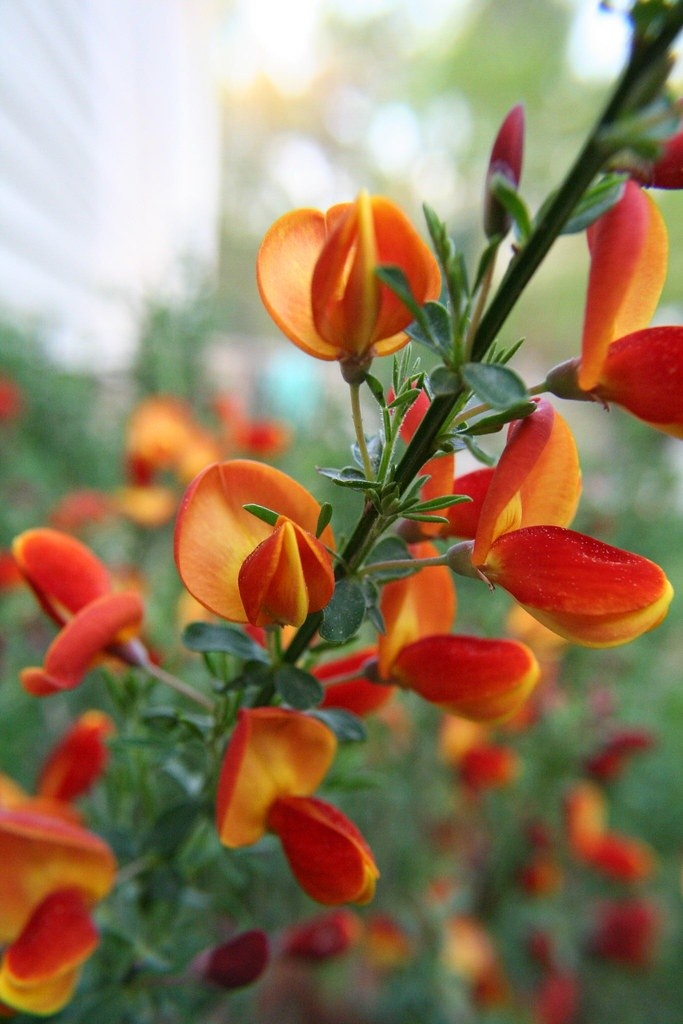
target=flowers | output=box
[0,0,683,1024]
